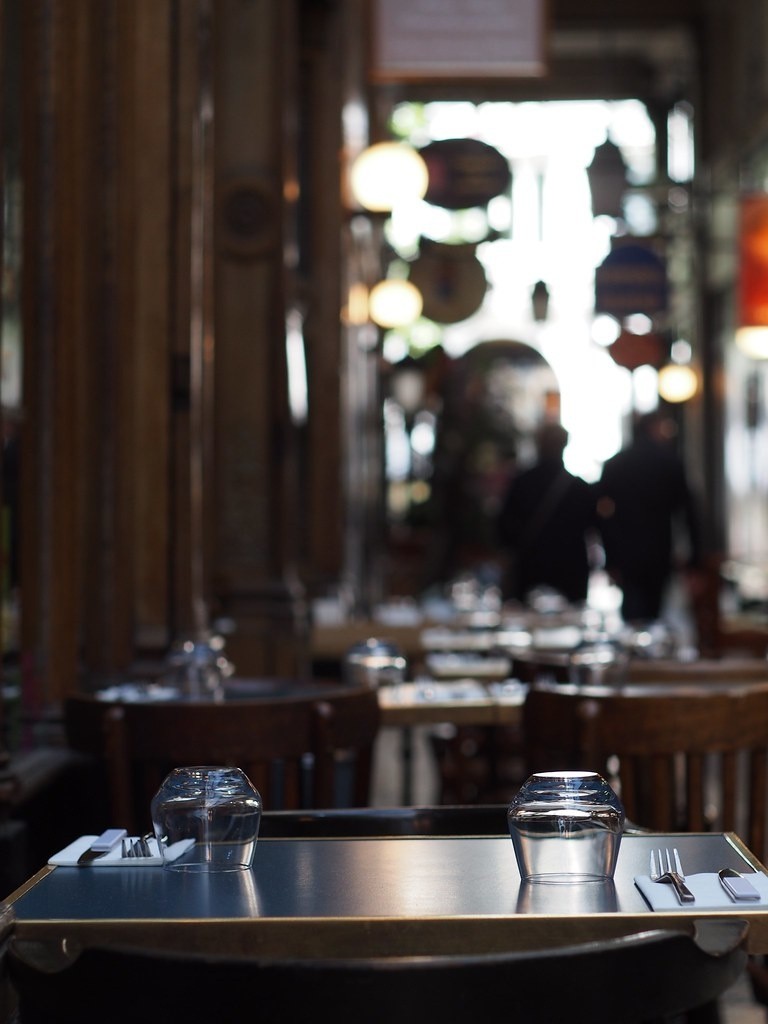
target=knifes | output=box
[719,868,760,903]
[78,829,127,865]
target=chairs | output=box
[60,697,333,829]
[4,919,755,1022]
[524,680,768,1011]
[333,690,379,813]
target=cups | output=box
[151,768,262,874]
[507,771,625,882]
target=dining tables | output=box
[379,703,524,811]
[0,826,768,1024]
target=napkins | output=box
[46,834,194,870]
[635,869,768,914]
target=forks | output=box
[122,833,152,857]
[650,848,697,905]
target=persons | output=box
[371,351,703,649]
